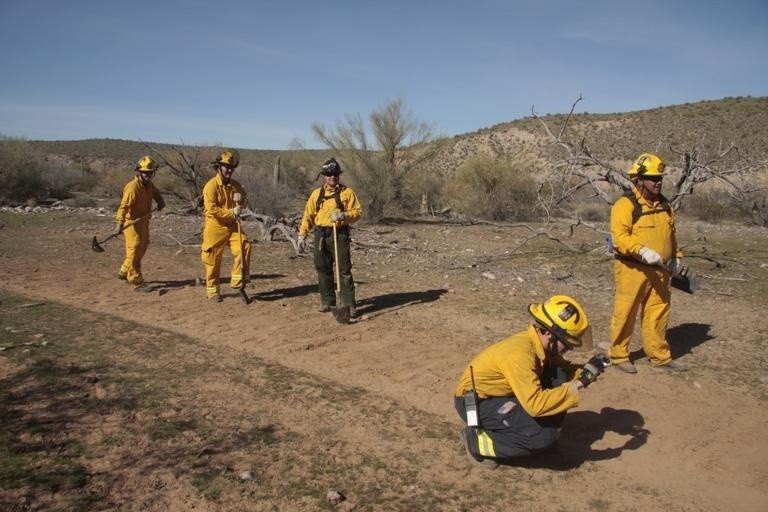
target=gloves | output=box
[580,352,605,386]
[232,205,242,215]
[639,247,664,267]
[157,199,166,210]
[329,212,344,222]
[113,223,123,239]
[297,238,305,252]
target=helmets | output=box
[320,159,342,174]
[526,296,590,349]
[135,155,158,172]
[218,150,239,168]
[627,154,667,175]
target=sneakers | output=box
[349,307,356,319]
[319,304,330,312]
[459,424,499,469]
[118,273,128,279]
[616,362,636,373]
[135,284,154,293]
[234,281,255,289]
[657,360,689,371]
[211,294,220,302]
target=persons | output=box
[608,151,689,375]
[453,294,612,472]
[296,154,364,323]
[114,153,168,293]
[200,149,253,303]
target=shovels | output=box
[92,207,160,252]
[656,262,693,295]
[329,221,350,324]
[236,201,250,305]
